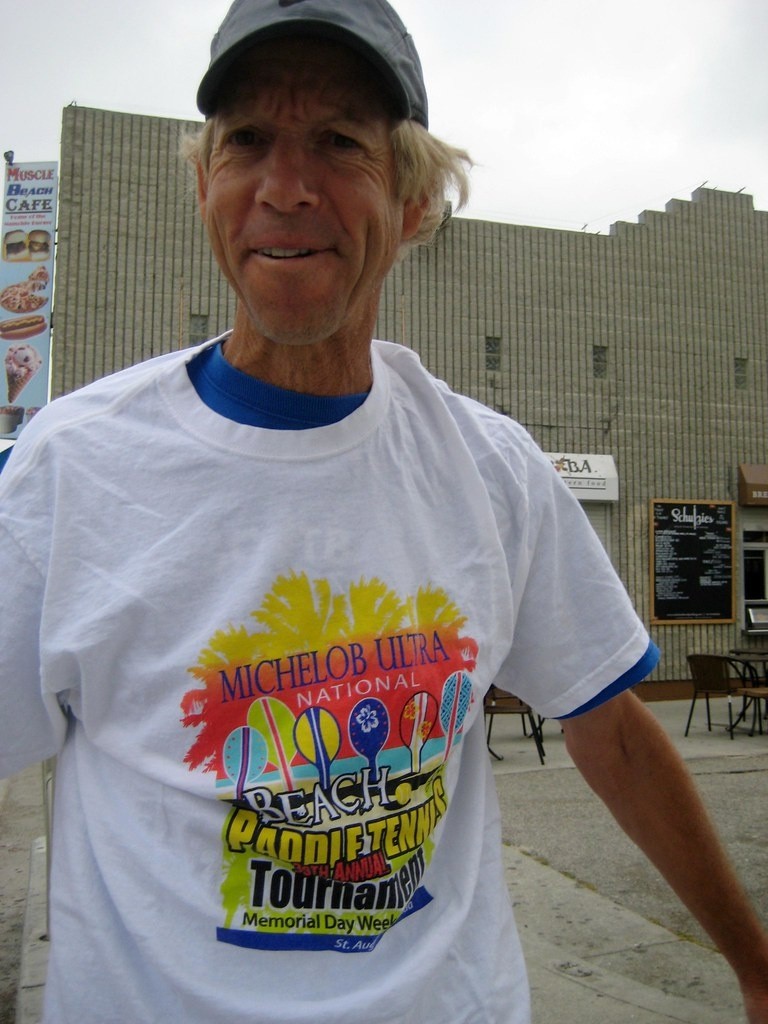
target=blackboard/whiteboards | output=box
[648,497,737,626]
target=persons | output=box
[0,1,767,1024]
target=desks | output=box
[730,655,768,721]
[729,648,768,697]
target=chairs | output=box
[684,654,759,739]
[725,688,768,738]
[482,683,546,765]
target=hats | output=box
[195,0,428,130]
